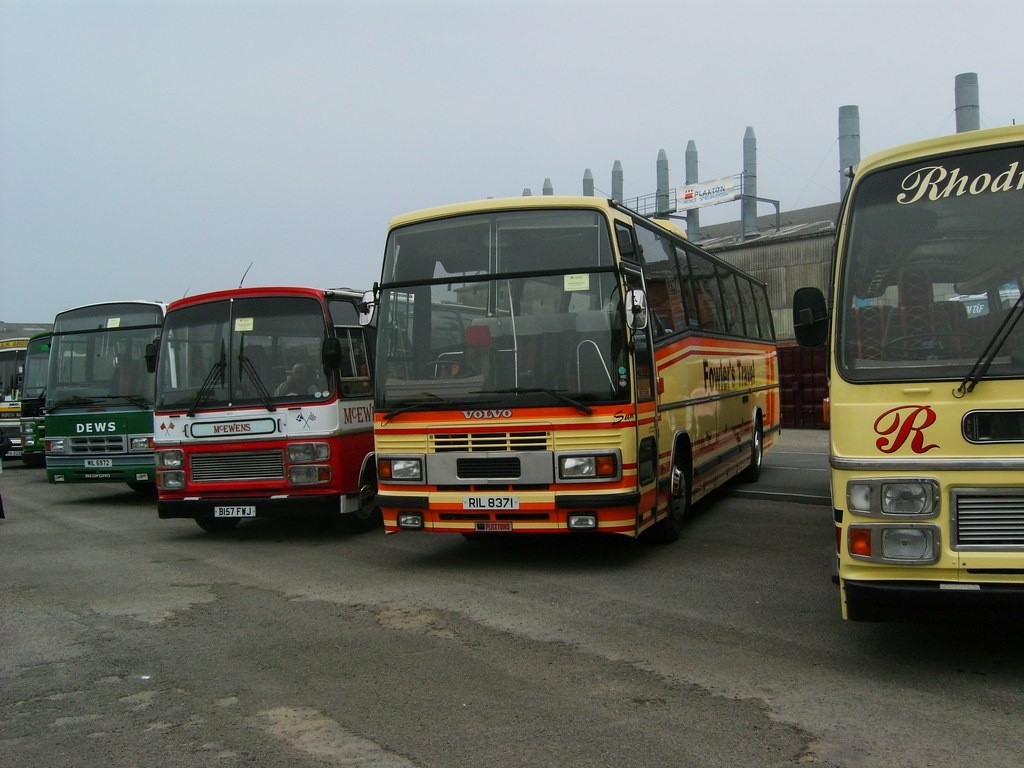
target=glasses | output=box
[293,370,304,376]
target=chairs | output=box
[226,341,312,398]
[473,309,610,374]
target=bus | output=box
[44,299,179,489]
[823,125,1024,630]
[359,194,784,540]
[0,333,144,464]
[145,287,516,538]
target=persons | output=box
[353,345,368,376]
[274,363,318,397]
